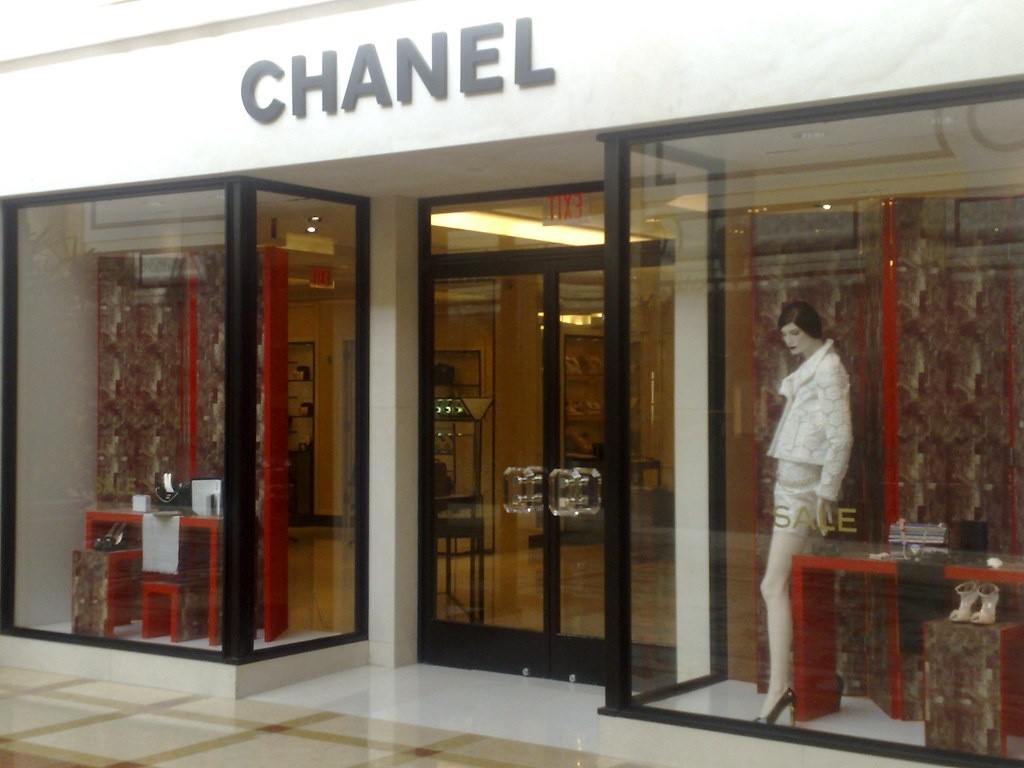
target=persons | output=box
[751,300,853,727]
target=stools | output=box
[140,576,209,642]
[71,547,142,639]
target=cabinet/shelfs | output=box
[557,328,644,534]
[434,348,484,558]
[288,342,315,527]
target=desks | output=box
[85,507,259,646]
[791,547,1024,723]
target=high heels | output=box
[102,520,130,551]
[93,521,122,551]
[947,582,980,622]
[969,581,1002,624]
[755,687,796,726]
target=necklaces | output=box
[155,486,179,503]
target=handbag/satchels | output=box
[154,480,191,508]
